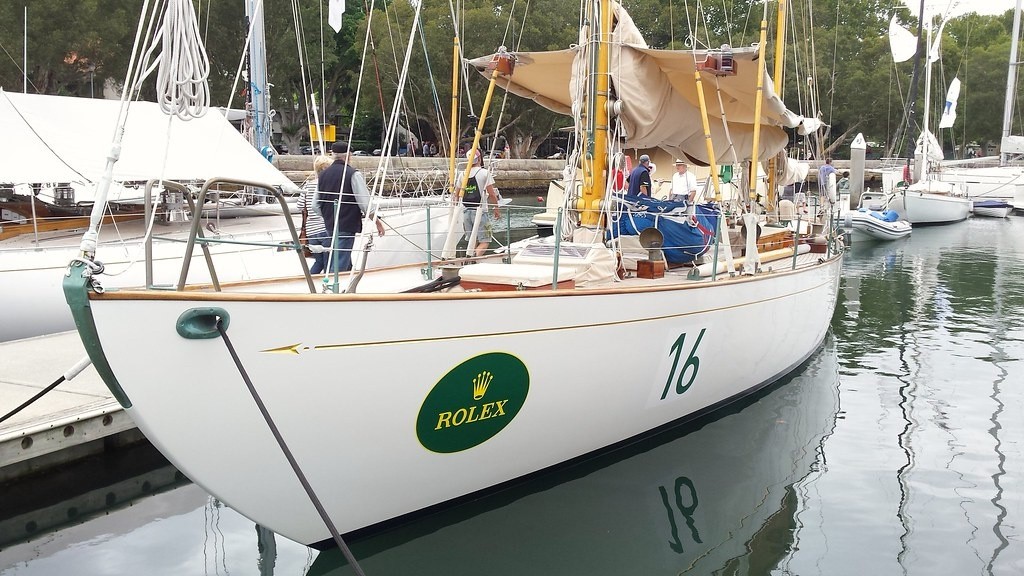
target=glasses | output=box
[650,166,652,169]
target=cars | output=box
[548,154,566,160]
[373,148,381,155]
[298,145,322,156]
[353,151,371,156]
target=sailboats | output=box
[62,0,844,555]
[0,0,465,344]
[908,0,1024,210]
[886,0,971,224]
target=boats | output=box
[838,187,912,241]
[973,200,1013,219]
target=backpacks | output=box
[459,167,483,208]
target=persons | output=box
[817,158,837,217]
[612,152,656,197]
[838,171,850,194]
[453,149,499,264]
[669,159,697,201]
[297,156,333,274]
[795,182,808,215]
[312,142,385,273]
[406,138,436,157]
[963,144,984,159]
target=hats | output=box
[649,164,656,176]
[640,153,648,162]
[332,141,352,153]
[466,149,480,164]
[673,158,692,168]
